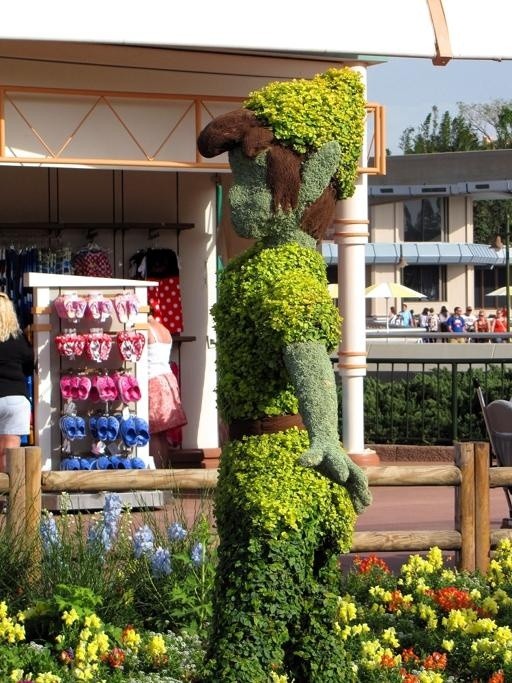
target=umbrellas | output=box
[366,280,430,336]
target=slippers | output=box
[56,293,150,469]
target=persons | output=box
[388,302,511,343]
[0,288,37,479]
[140,297,190,466]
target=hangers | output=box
[0,229,166,257]
